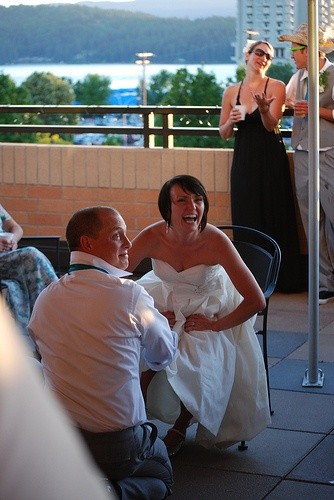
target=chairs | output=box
[0,235,60,294]
[216,225,282,450]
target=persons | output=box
[121,175,274,458]
[0,204,59,322]
[219,42,304,292]
[277,23,334,305]
[28,205,178,500]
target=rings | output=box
[192,322,195,325]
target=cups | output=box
[234,105,247,121]
[293,100,306,117]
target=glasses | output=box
[254,48,271,61]
[288,46,306,54]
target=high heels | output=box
[164,417,190,458]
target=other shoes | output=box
[319,290,334,299]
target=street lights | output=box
[135,52,154,146]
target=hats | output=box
[280,22,334,54]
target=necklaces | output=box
[245,78,263,102]
